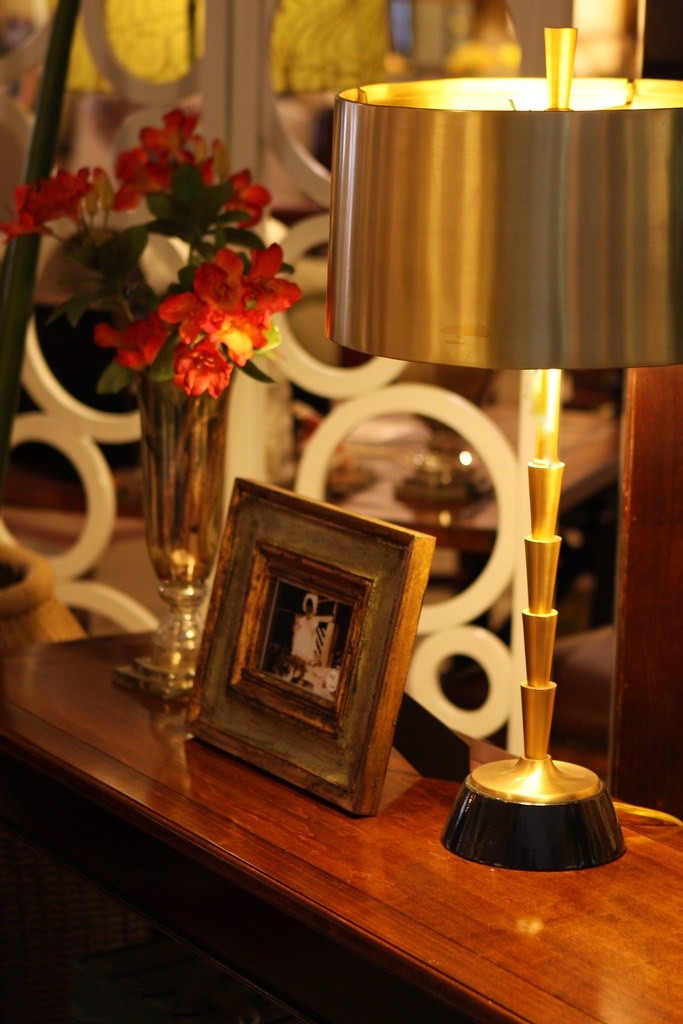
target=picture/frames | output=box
[182,476,438,821]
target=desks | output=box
[0,629,683,1024]
[331,409,617,668]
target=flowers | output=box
[0,101,304,550]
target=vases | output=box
[113,370,239,703]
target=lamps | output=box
[327,26,683,871]
